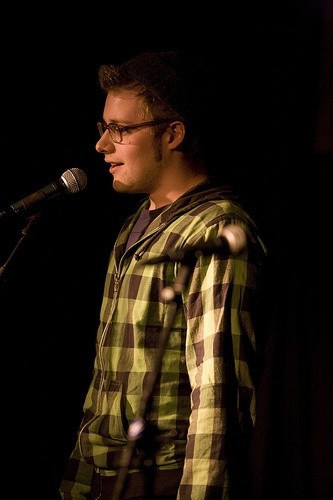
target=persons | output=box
[57,52,274,499]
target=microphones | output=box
[1,168,88,218]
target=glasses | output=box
[96,118,168,144]
